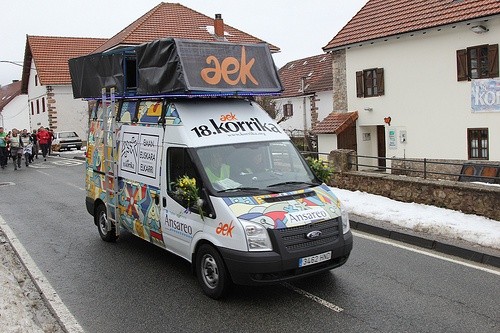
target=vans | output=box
[87,99,352,300]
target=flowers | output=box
[304,156,336,183]
[171,173,205,222]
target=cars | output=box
[52,131,82,150]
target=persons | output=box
[0,126,54,170]
